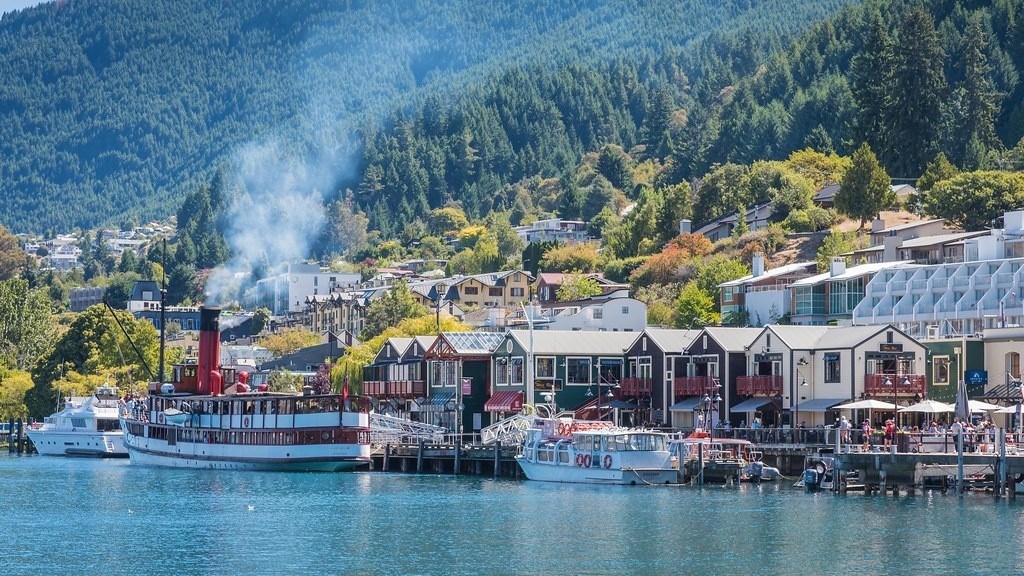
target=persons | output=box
[707,418,775,443]
[798,421,806,428]
[913,417,997,452]
[831,416,855,444]
[882,416,895,453]
[862,420,870,453]
[246,401,255,414]
[117,394,150,419]
[204,433,219,444]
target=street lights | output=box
[701,374,723,444]
[795,368,808,443]
[881,354,910,448]
[606,369,621,422]
[584,372,615,421]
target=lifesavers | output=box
[603,454,613,469]
[158,413,164,423]
[244,416,249,428]
[575,453,584,467]
[583,454,591,469]
[558,421,602,437]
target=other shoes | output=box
[864,449,870,453]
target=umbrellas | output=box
[596,399,643,428]
[954,381,970,421]
[832,400,1024,437]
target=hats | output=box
[865,419,870,422]
[842,415,845,420]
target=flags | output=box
[341,369,349,401]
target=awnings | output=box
[669,397,706,412]
[484,391,524,412]
[420,392,455,412]
[791,398,850,412]
[730,396,782,413]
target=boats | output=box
[25,372,130,457]
[117,307,373,473]
[664,435,762,483]
[514,431,688,485]
[831,448,1024,493]
